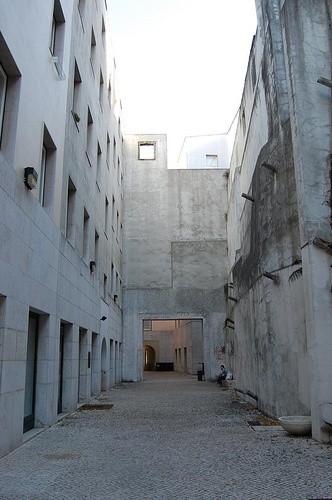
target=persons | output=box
[216,364,228,387]
